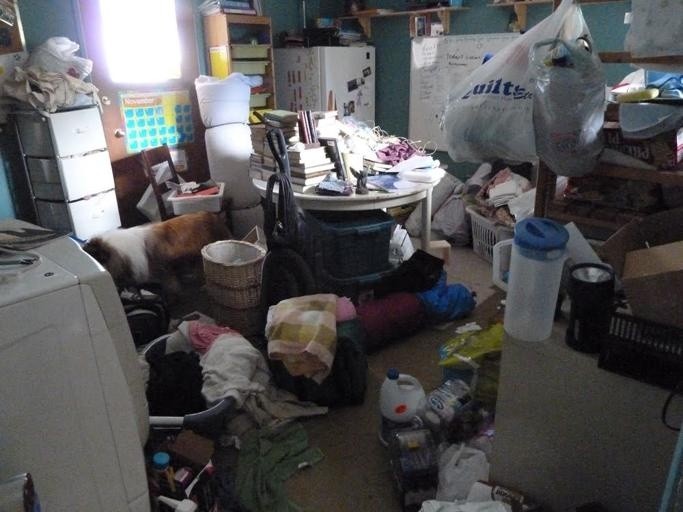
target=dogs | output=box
[81,209,234,291]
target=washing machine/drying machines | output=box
[0,238,150,510]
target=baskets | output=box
[597,300,683,394]
[304,209,395,280]
[201,239,266,337]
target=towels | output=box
[267,292,358,384]
[488,181,523,207]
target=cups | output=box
[493,217,570,344]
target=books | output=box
[362,155,392,173]
[198,1,257,15]
[0,218,73,255]
[249,110,348,192]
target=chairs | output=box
[141,143,236,237]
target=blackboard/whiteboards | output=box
[408,33,524,152]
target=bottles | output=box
[412,378,472,431]
[150,452,171,475]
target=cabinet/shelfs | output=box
[534,155,683,244]
[200,14,277,128]
[12,105,123,245]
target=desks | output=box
[489,304,682,512]
[253,168,447,257]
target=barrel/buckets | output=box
[378,368,426,446]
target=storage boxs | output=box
[621,240,683,331]
[166,182,225,215]
[601,98,683,167]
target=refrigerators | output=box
[275,45,376,125]
